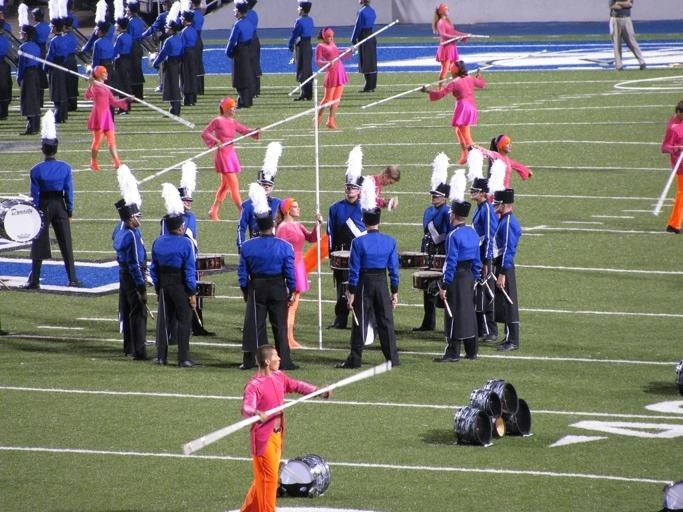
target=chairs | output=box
[666,225,681,233]
[617,64,647,71]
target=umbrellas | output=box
[67,279,82,286]
[18,283,40,289]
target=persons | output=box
[333,173,400,369]
[150,182,202,369]
[311,26,355,130]
[237,182,299,369]
[274,197,322,349]
[202,96,261,220]
[608,0,648,70]
[238,141,283,254]
[663,100,683,234]
[240,343,330,512]
[159,160,216,345]
[351,0,378,92]
[470,133,532,204]
[433,167,482,363]
[327,143,366,328]
[432,2,470,91]
[412,153,456,333]
[370,168,400,209]
[487,158,520,352]
[113,163,158,361]
[19,137,82,290]
[87,65,136,172]
[467,147,499,343]
[0,4,260,137]
[288,1,314,102]
[422,59,487,164]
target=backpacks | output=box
[0,198,46,243]
[330,250,350,270]
[278,453,330,497]
[429,255,446,271]
[195,255,224,271]
[453,378,531,446]
[195,281,215,297]
[398,252,430,268]
[663,480,682,512]
[412,270,443,289]
